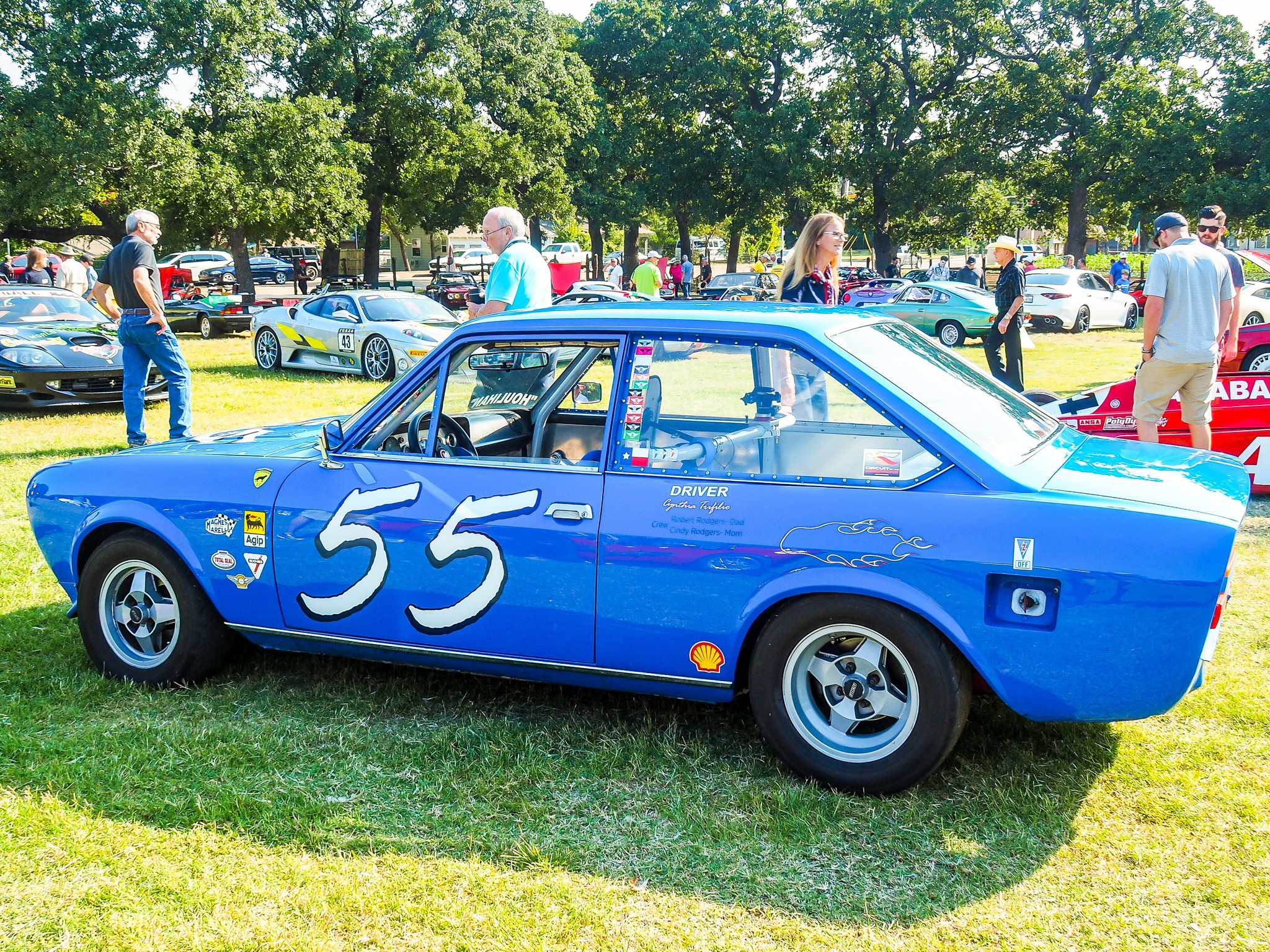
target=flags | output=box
[446,247,456,272]
[1133,222,1140,245]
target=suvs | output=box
[1014,243,1044,266]
[156,250,235,282]
[262,246,322,281]
[0,253,63,280]
[427,246,500,276]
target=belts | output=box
[998,305,1022,312]
[123,310,155,315]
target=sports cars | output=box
[163,290,283,339]
[424,270,482,309]
[248,288,464,382]
[839,269,1032,347]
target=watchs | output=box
[1004,315,1010,320]
[474,309,479,319]
[1141,347,1152,354]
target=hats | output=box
[670,257,679,263]
[1022,257,1033,263]
[5,255,14,260]
[80,254,92,262]
[57,246,79,256]
[777,258,782,263]
[1119,252,1127,259]
[984,235,1023,255]
[966,256,975,264]
[300,260,308,264]
[647,250,662,258]
[1147,212,1188,249]
[763,253,771,260]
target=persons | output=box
[1197,204,1245,362]
[985,235,1026,393]
[261,248,270,258]
[297,260,311,295]
[778,213,845,421]
[93,209,193,448]
[0,246,98,319]
[1132,212,1237,451]
[883,256,902,278]
[884,252,1130,300]
[602,251,785,298]
[574,385,589,402]
[463,206,559,410]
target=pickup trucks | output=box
[542,242,592,264]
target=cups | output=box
[468,287,485,305]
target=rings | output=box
[1232,356,1234,357]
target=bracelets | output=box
[1006,313,1012,319]
[778,376,790,382]
[115,317,122,323]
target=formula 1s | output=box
[1013,368,1270,499]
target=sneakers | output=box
[129,438,155,449]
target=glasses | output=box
[1120,258,1126,259]
[1197,224,1223,233]
[823,231,849,242]
[481,225,512,241]
[137,221,160,230]
[611,261,616,263]
[1153,231,1161,247]
[969,262,975,266]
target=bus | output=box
[675,236,727,263]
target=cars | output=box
[771,248,790,269]
[603,251,648,265]
[24,295,1255,793]
[309,275,371,296]
[1128,279,1148,315]
[1218,276,1270,375]
[0,273,11,286]
[1021,268,1139,334]
[836,266,884,303]
[198,256,295,288]
[685,272,780,302]
[550,280,665,304]
[659,272,676,300]
[0,283,168,412]
